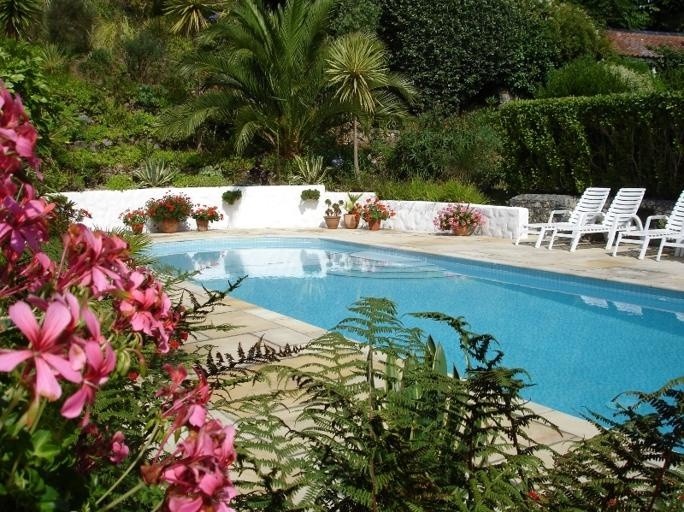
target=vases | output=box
[157,219,180,233]
[453,226,475,236]
[196,218,208,231]
[130,224,144,234]
[342,214,360,229]
[368,218,380,230]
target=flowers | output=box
[118,208,148,225]
[361,198,395,222]
[144,190,193,223]
[190,203,223,223]
[432,203,486,231]
[344,192,362,215]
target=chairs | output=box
[514,188,683,261]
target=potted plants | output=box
[323,199,343,229]
